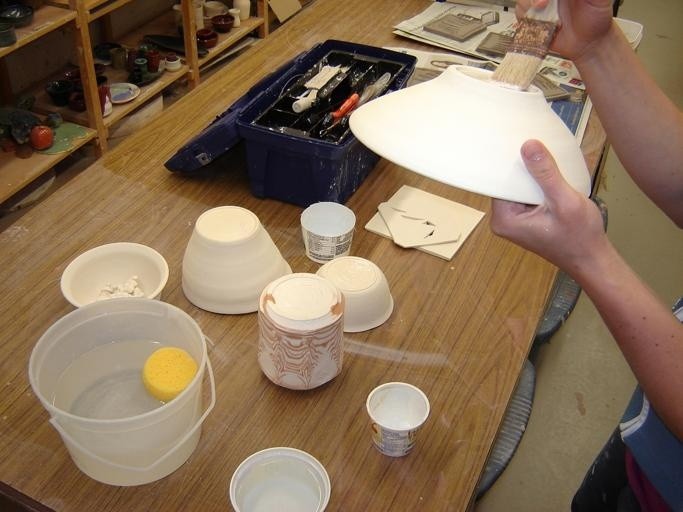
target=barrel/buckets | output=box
[28,296,216,488]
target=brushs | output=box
[490,1,564,94]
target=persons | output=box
[489,1,683,511]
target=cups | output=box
[173,4,204,36]
[144,51,160,73]
[164,56,182,72]
[365,382,431,459]
[109,48,126,69]
[228,8,241,29]
[0,22,17,48]
[44,79,73,107]
[69,91,87,113]
[258,273,345,391]
[301,201,357,264]
[228,447,332,512]
[205,2,228,16]
[181,206,293,315]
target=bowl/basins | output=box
[61,242,169,308]
[1,5,34,28]
[348,63,593,208]
[93,41,122,60]
[315,256,394,334]
[212,15,234,33]
[63,63,106,90]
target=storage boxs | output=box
[164,40,417,208]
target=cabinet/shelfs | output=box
[0,1,273,213]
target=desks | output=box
[1,1,635,512]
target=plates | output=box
[144,33,210,56]
[107,82,141,104]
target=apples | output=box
[30,126,52,150]
